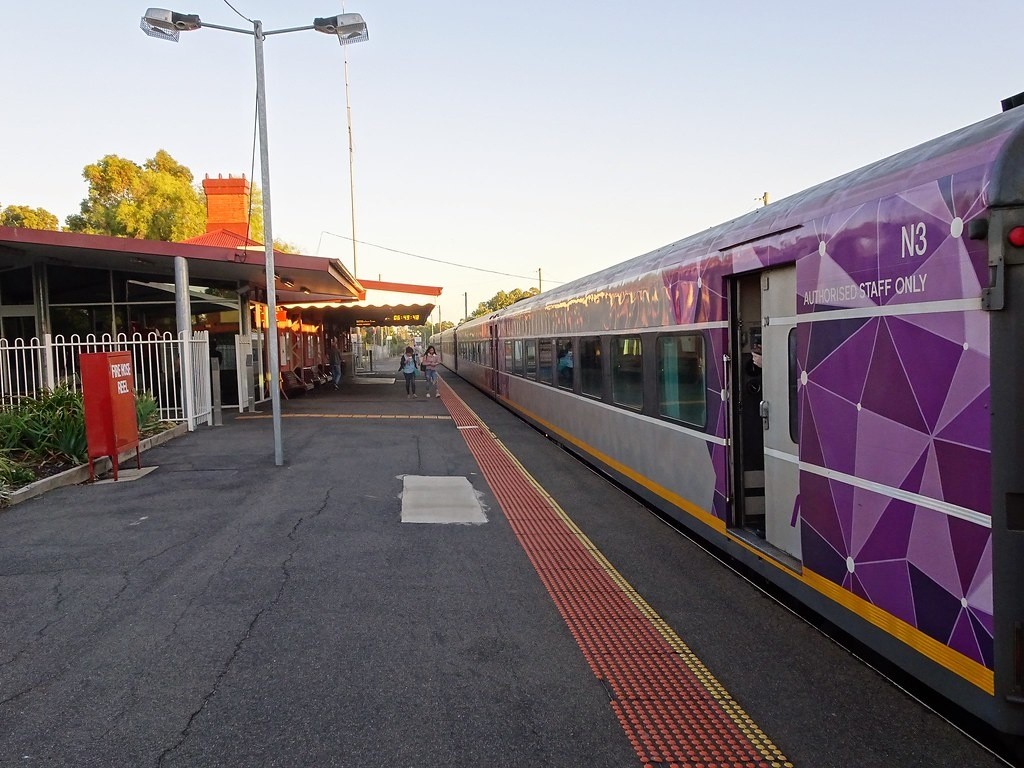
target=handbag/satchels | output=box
[421,355,426,370]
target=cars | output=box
[527,356,536,366]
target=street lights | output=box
[139,6,369,466]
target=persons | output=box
[752,352,762,367]
[557,342,573,382]
[329,343,344,393]
[398,346,419,399]
[422,345,441,398]
[413,345,417,352]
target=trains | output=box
[420,94,1024,747]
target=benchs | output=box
[281,364,333,398]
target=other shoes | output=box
[333,383,339,390]
[436,394,441,398]
[407,395,410,399]
[426,393,430,398]
[413,394,418,398]
